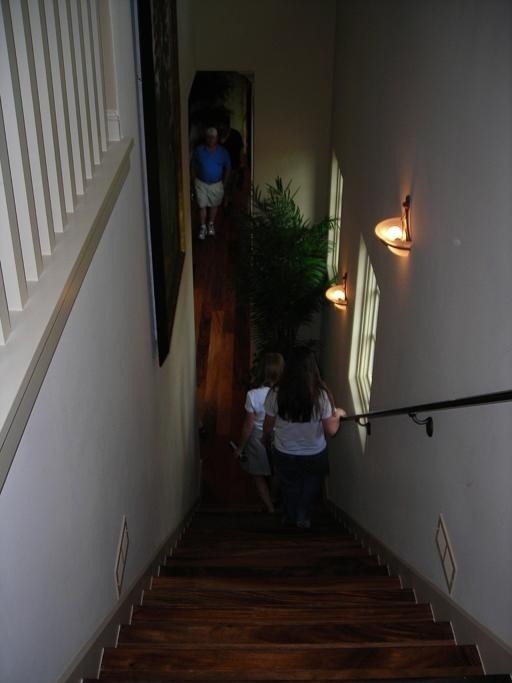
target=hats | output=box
[207,127,218,137]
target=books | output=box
[230,441,246,457]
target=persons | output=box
[191,126,232,239]
[264,343,348,527]
[231,352,285,514]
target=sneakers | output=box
[199,225,208,239]
[208,222,215,236]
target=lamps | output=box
[325,271,348,310]
[372,195,413,257]
